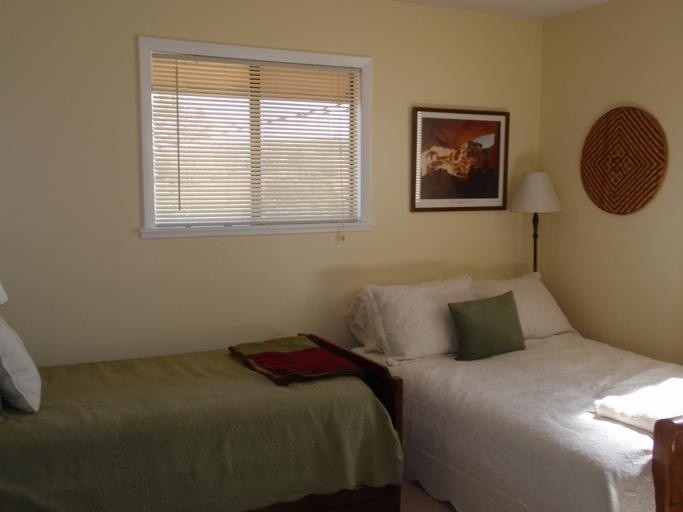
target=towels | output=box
[591,375,682,433]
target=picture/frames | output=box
[410,106,509,212]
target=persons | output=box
[466,147,497,198]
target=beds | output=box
[1,333,406,512]
[349,330,683,511]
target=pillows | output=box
[0,283,45,416]
[363,270,577,363]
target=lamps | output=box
[511,172,562,275]
